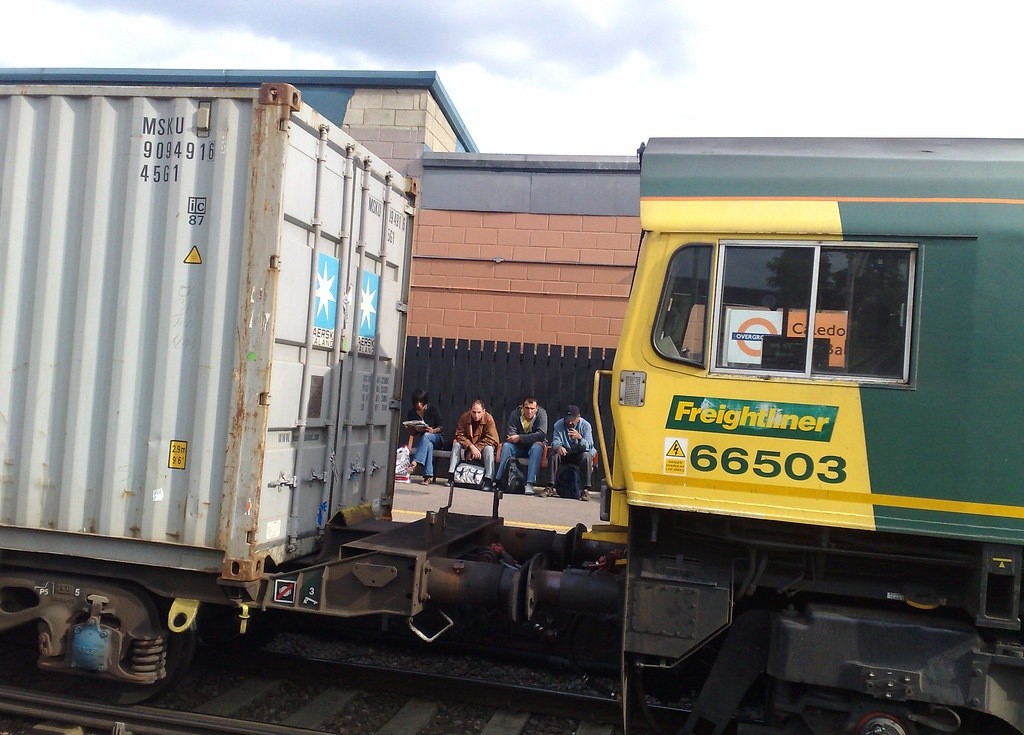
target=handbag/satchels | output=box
[395,443,412,484]
[453,462,485,489]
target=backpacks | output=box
[555,464,582,499]
[498,456,527,493]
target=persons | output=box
[406,389,448,485]
[493,398,547,494]
[539,404,597,502]
[445,400,499,492]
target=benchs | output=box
[407,448,593,488]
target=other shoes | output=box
[482,478,493,491]
[539,485,555,497]
[524,482,534,495]
[407,464,418,472]
[420,476,433,485]
[492,484,510,491]
[579,490,590,501]
[445,473,455,486]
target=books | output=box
[402,420,429,433]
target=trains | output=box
[0,72,1023,734]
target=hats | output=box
[564,405,580,421]
